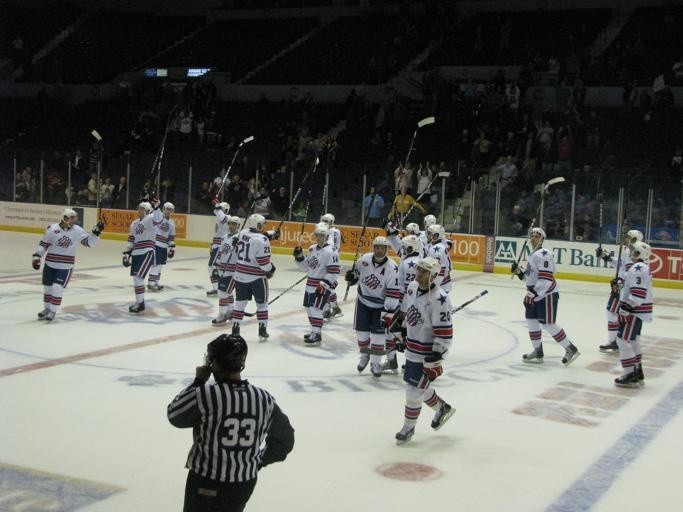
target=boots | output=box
[38,308,51,317]
[431,402,452,429]
[304,306,342,344]
[370,360,383,378]
[614,363,645,385]
[207,290,218,295]
[522,347,546,360]
[356,352,371,372]
[212,317,227,323]
[258,322,270,338]
[46,311,56,321]
[599,339,620,351]
[129,283,164,313]
[396,424,415,441]
[378,356,400,370]
[562,341,579,365]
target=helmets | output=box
[401,214,445,255]
[530,228,549,242]
[206,331,250,374]
[626,230,645,246]
[247,214,267,231]
[139,202,151,211]
[629,242,652,261]
[416,257,442,276]
[163,202,175,212]
[314,221,330,238]
[227,216,240,228]
[320,212,336,225]
[220,202,231,213]
[372,235,390,247]
[59,208,78,224]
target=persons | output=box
[345,215,456,446]
[167,334,295,512]
[205,201,275,340]
[122,201,175,314]
[510,227,581,365]
[1,64,683,246]
[291,213,343,347]
[600,230,653,388]
[32,209,107,321]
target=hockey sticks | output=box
[344,181,387,302]
[243,276,307,317]
[511,176,565,278]
[398,172,451,226]
[322,141,337,214]
[214,136,255,198]
[616,165,638,284]
[384,117,433,228]
[360,290,490,355]
[149,104,183,199]
[275,143,320,234]
[91,130,102,227]
